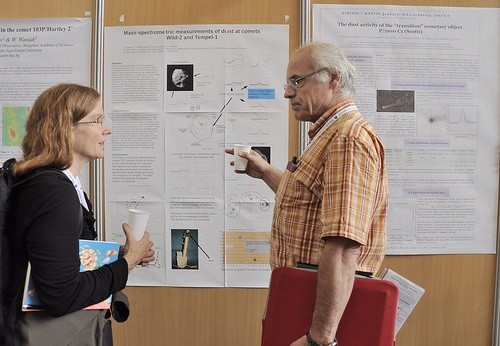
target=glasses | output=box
[73,116,104,126]
[283,67,327,92]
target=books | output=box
[21,237,120,311]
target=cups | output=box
[128,209,150,241]
[234,143,252,170]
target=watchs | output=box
[306,331,338,346]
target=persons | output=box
[225,42,389,346]
[0,83,156,346]
